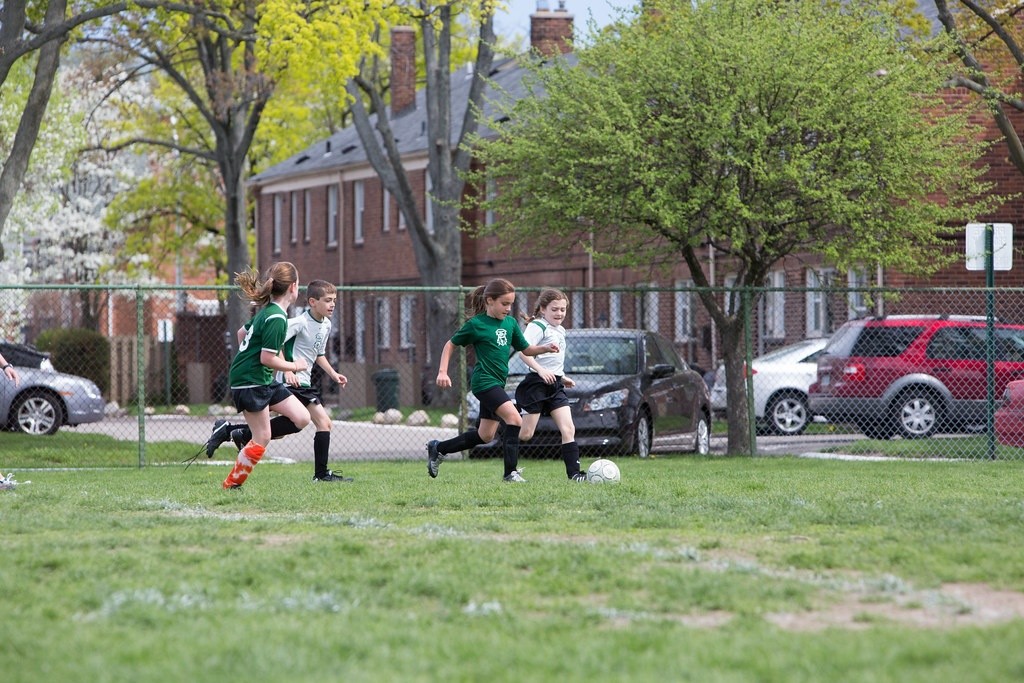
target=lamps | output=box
[596,311,610,329]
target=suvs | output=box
[806,313,1024,440]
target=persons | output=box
[223,261,311,489]
[0,354,19,386]
[425,279,560,482]
[518,287,587,482]
[206,280,355,484]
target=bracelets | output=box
[294,362,298,372]
[3,363,13,371]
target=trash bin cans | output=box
[371,367,400,413]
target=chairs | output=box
[570,354,592,367]
[603,355,655,373]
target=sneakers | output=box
[182,420,231,471]
[425,440,445,478]
[570,472,587,482]
[222,481,242,493]
[313,470,354,483]
[503,471,526,482]
[230,429,247,452]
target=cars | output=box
[994,380,1024,448]
[0,366,106,436]
[458,327,713,458]
[0,343,54,374]
[709,337,830,436]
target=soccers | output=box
[587,458,621,484]
